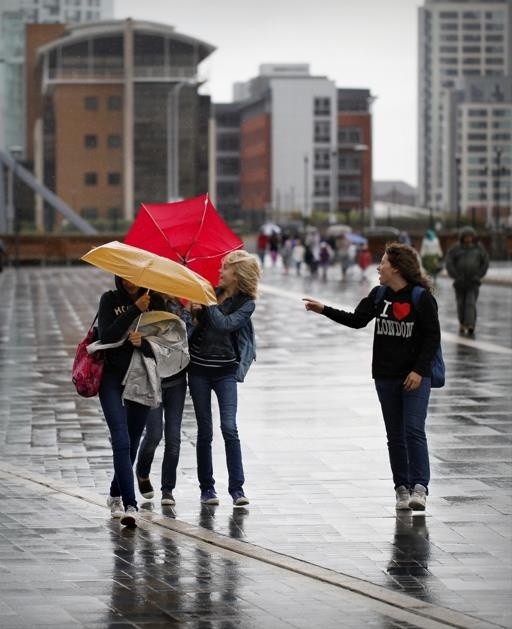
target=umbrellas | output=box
[122,192,246,307]
[78,241,218,335]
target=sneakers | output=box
[233,491,249,505]
[161,491,175,505]
[120,504,139,526]
[107,495,124,517]
[136,473,154,498]
[200,489,219,504]
[395,485,412,510]
[408,483,426,511]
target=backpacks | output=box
[233,296,256,383]
[374,286,446,388]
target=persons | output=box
[135,291,199,507]
[256,219,443,287]
[98,273,165,529]
[301,243,439,512]
[445,226,490,334]
[187,250,261,508]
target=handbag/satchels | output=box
[71,291,116,397]
